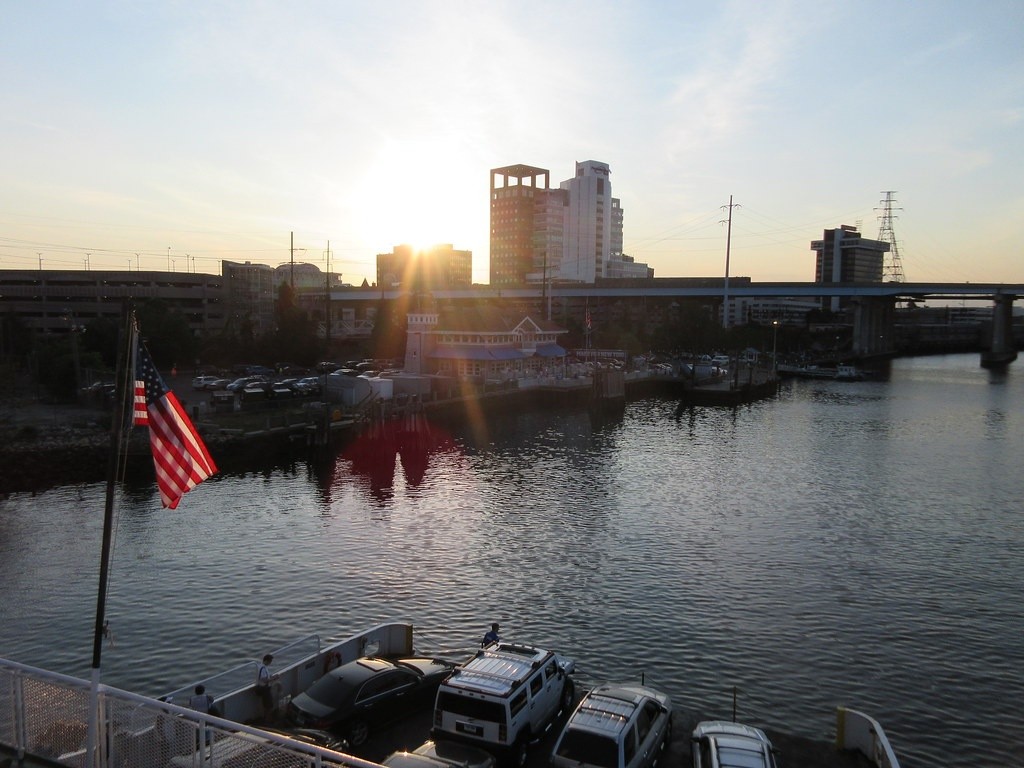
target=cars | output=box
[383,739,497,768]
[227,366,319,396]
[646,363,673,374]
[207,379,231,389]
[691,720,778,768]
[344,358,399,373]
[284,654,457,749]
[168,725,348,768]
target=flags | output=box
[586,308,592,330]
[130,321,220,511]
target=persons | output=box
[481,623,500,648]
[190,685,214,750]
[256,655,280,724]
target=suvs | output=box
[192,375,220,390]
[547,680,674,768]
[430,638,575,768]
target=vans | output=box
[712,356,729,365]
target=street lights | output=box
[414,331,421,373]
[773,321,778,372]
